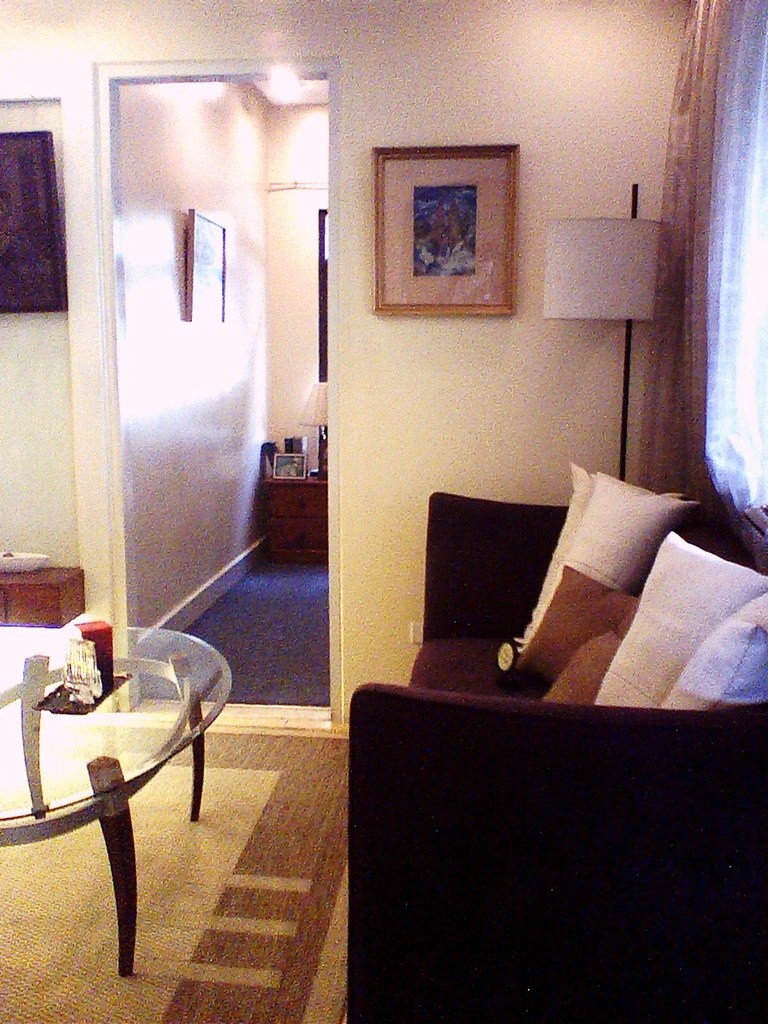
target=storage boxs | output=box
[0,569,84,627]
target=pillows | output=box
[514,462,698,686]
[540,630,623,706]
[522,563,640,688]
[594,531,768,709]
[662,592,767,709]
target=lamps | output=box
[544,182,672,492]
[300,382,332,481]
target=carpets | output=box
[0,718,349,1021]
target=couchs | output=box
[349,495,768,1024]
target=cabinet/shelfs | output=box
[265,478,331,565]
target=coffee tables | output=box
[0,619,233,980]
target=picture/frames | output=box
[273,454,306,480]
[371,143,524,320]
